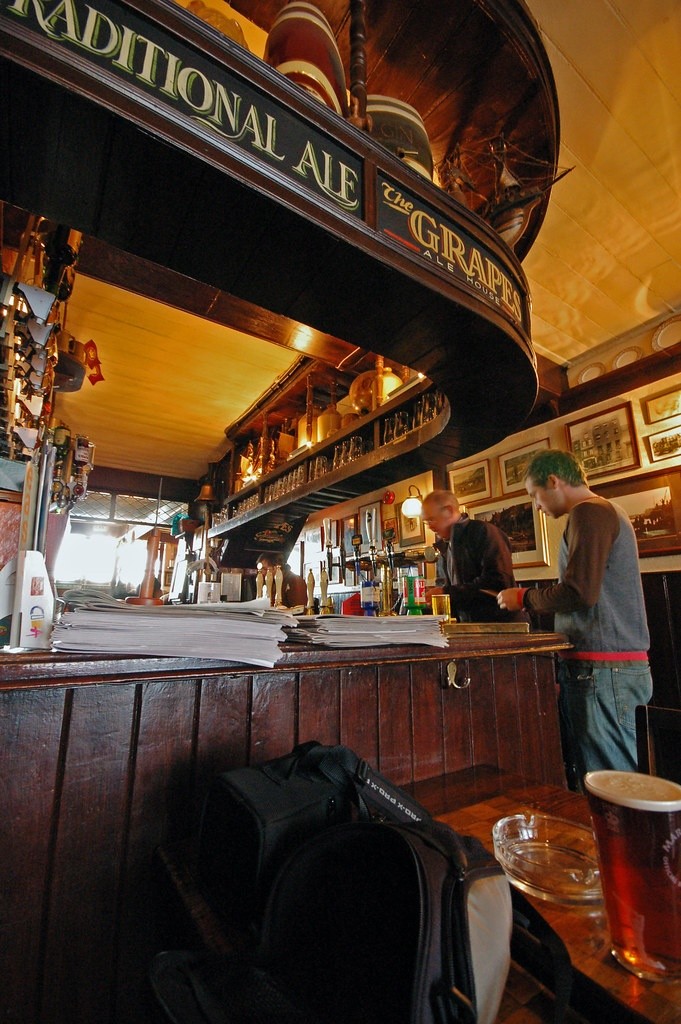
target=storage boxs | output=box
[176,519,199,533]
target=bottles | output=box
[88,442,95,471]
[215,507,227,527]
[211,573,216,583]
[317,404,341,443]
[73,434,88,477]
[54,426,71,477]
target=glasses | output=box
[422,505,446,525]
[258,567,275,573]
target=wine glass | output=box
[402,576,426,615]
[361,581,380,617]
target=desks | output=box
[391,763,681,1024]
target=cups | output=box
[432,595,451,634]
[264,466,307,503]
[384,391,449,446]
[584,770,681,982]
[309,437,373,482]
[232,493,259,519]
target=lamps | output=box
[400,485,423,518]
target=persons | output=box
[633,497,668,532]
[423,490,516,623]
[257,551,307,615]
[134,575,162,598]
[425,578,445,608]
[495,449,654,785]
[512,463,518,479]
[652,433,681,452]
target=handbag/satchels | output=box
[139,740,515,1024]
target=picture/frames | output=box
[325,520,338,549]
[341,513,358,556]
[397,560,426,580]
[397,494,426,547]
[498,436,550,495]
[645,388,681,425]
[286,541,303,576]
[356,562,368,585]
[358,500,383,555]
[305,526,323,553]
[302,561,322,587]
[383,518,399,544]
[564,400,643,480]
[344,559,356,586]
[458,488,550,569]
[588,464,681,559]
[368,568,381,583]
[165,570,173,586]
[647,426,681,463]
[448,458,492,505]
[323,556,342,584]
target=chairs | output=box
[634,704,681,784]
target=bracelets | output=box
[517,587,528,610]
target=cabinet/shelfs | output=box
[208,353,451,537]
